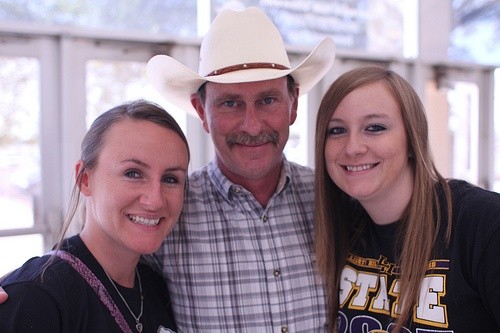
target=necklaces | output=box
[97,258,144,333]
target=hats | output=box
[145,7,335,117]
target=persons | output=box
[0,8,335,333]
[310,67,500,333]
[0,99,192,333]
